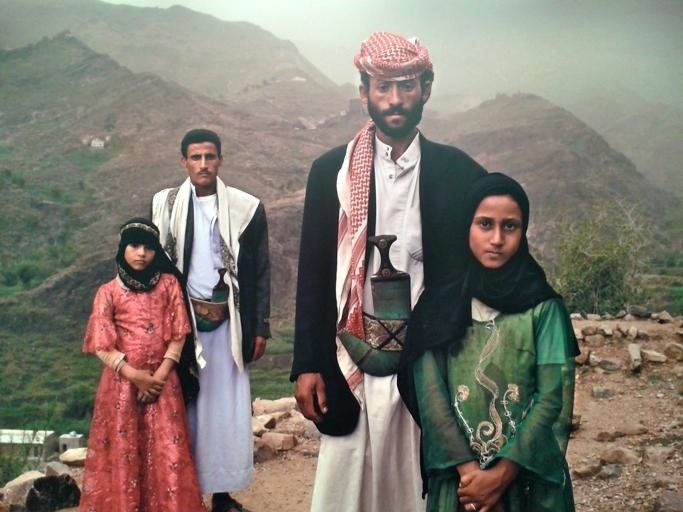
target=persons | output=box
[395,172,582,511]
[289,30,486,512]
[150,129,269,510]
[78,218,205,512]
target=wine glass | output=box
[470,503,477,510]
